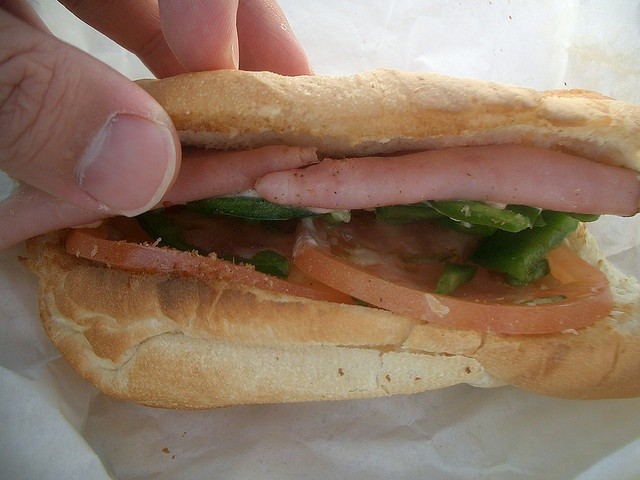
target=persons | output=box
[0,0,315,217]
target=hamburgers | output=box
[14,66,640,413]
